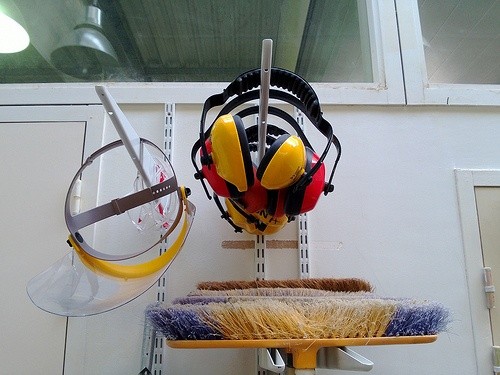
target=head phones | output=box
[189,67,342,235]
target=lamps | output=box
[0,0,31,54]
[49,0,121,81]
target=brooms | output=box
[145,274,452,375]
